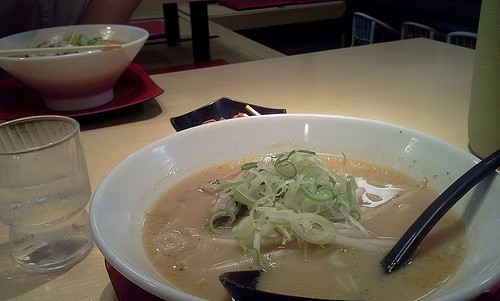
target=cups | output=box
[0,115,94,273]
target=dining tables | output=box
[0,37,500,301]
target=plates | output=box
[170,96,286,133]
[0,62,165,121]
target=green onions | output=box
[26,29,105,56]
[204,150,361,260]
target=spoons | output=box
[218,149,500,301]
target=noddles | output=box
[142,149,467,301]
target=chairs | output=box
[351,12,394,46]
[401,22,439,40]
[447,31,477,50]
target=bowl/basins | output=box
[0,24,150,111]
[89,114,499,301]
